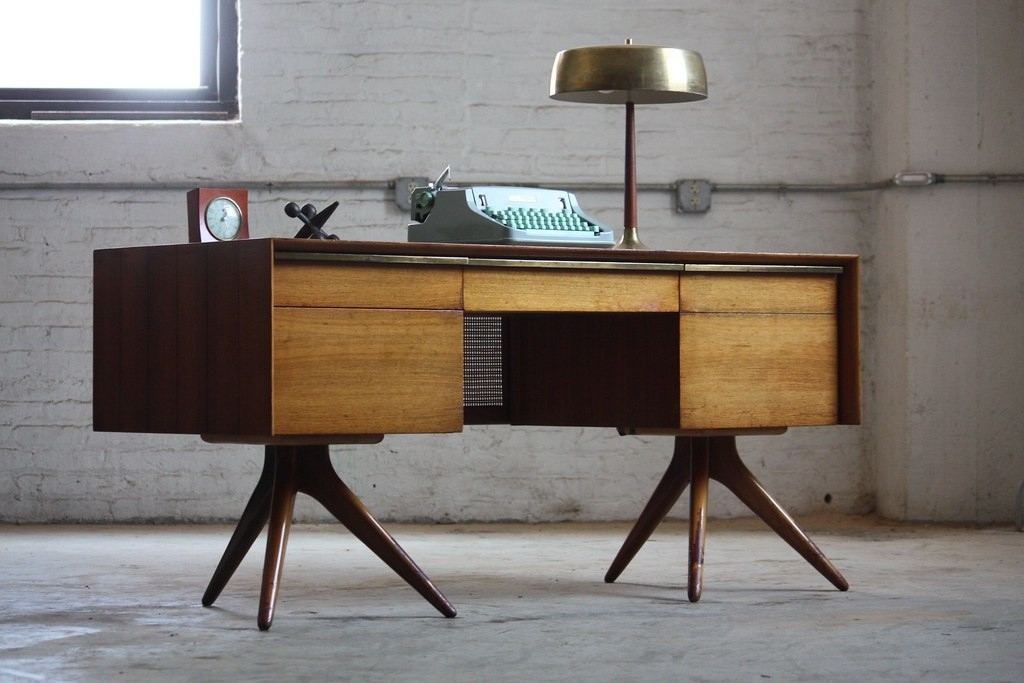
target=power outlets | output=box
[393,177,427,212]
[677,178,712,212]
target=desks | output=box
[91,237,865,632]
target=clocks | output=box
[186,186,249,243]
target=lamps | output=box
[549,37,707,251]
